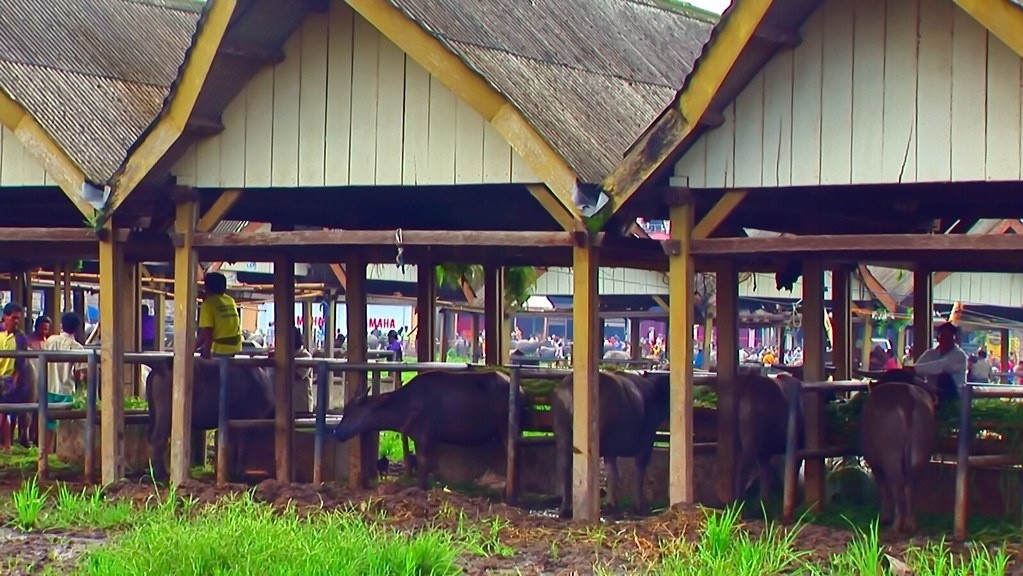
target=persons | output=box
[603,334,632,360]
[293,327,313,413]
[337,329,347,352]
[0,302,86,454]
[316,324,323,350]
[478,329,486,359]
[511,325,572,361]
[193,272,242,359]
[870,321,1023,422]
[456,332,472,358]
[694,340,717,369]
[640,333,666,361]
[371,325,410,385]
[738,342,804,367]
[242,329,274,351]
[142,304,155,347]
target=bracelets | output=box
[194,338,201,347]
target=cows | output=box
[553,372,670,515]
[855,367,948,536]
[737,365,803,504]
[344,372,524,495]
[146,359,276,482]
